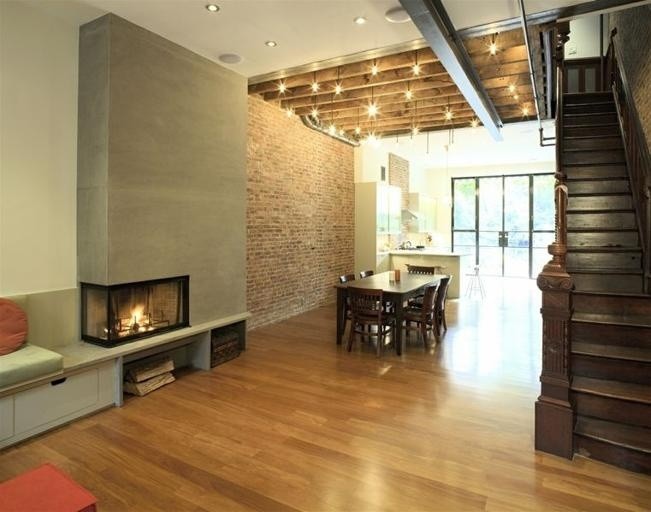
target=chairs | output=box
[333,265,452,359]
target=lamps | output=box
[279,32,530,147]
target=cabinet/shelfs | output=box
[1,312,252,451]
[409,193,438,245]
[355,181,403,312]
[388,252,460,299]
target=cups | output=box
[389,269,400,282]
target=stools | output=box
[0,464,98,512]
